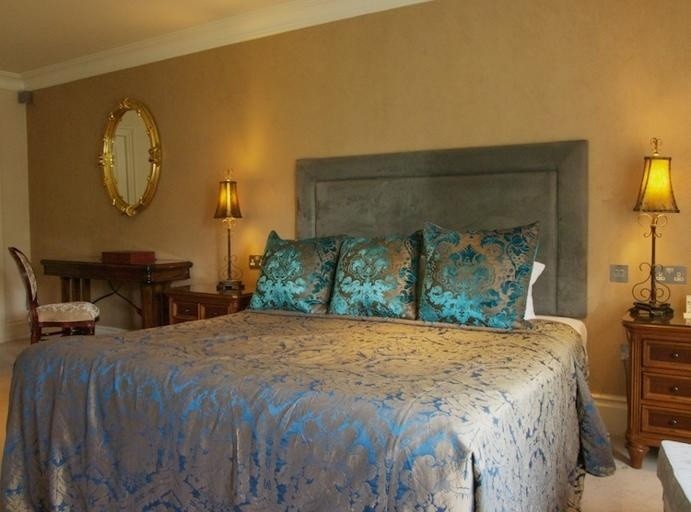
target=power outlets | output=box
[655,266,688,285]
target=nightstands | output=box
[622,303,691,469]
[164,282,253,324]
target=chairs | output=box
[7,246,99,344]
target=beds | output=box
[0,134,612,512]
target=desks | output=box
[39,258,191,332]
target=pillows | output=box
[419,254,547,322]
[327,230,423,320]
[246,230,346,313]
[416,221,541,331]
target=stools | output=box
[656,439,690,512]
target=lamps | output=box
[631,136,681,320]
[213,168,243,296]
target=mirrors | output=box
[100,97,160,219]
